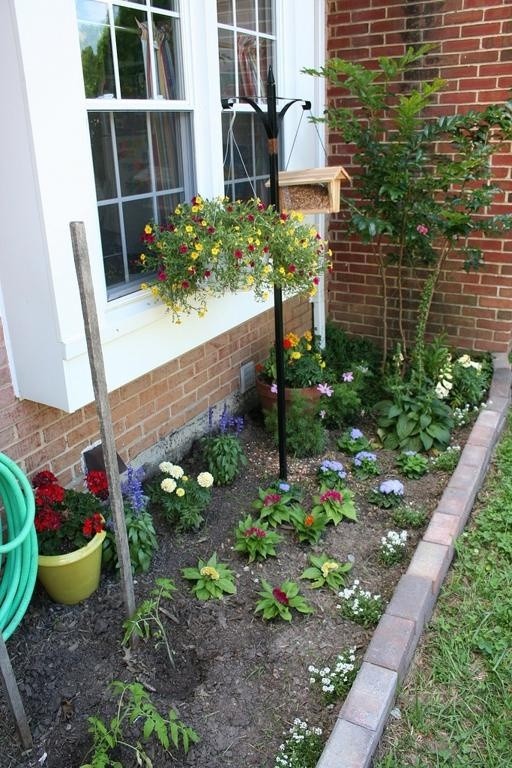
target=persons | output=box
[101,120,165,287]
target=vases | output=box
[258,377,317,418]
[39,531,105,606]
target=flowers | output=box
[253,327,328,386]
[27,464,111,557]
